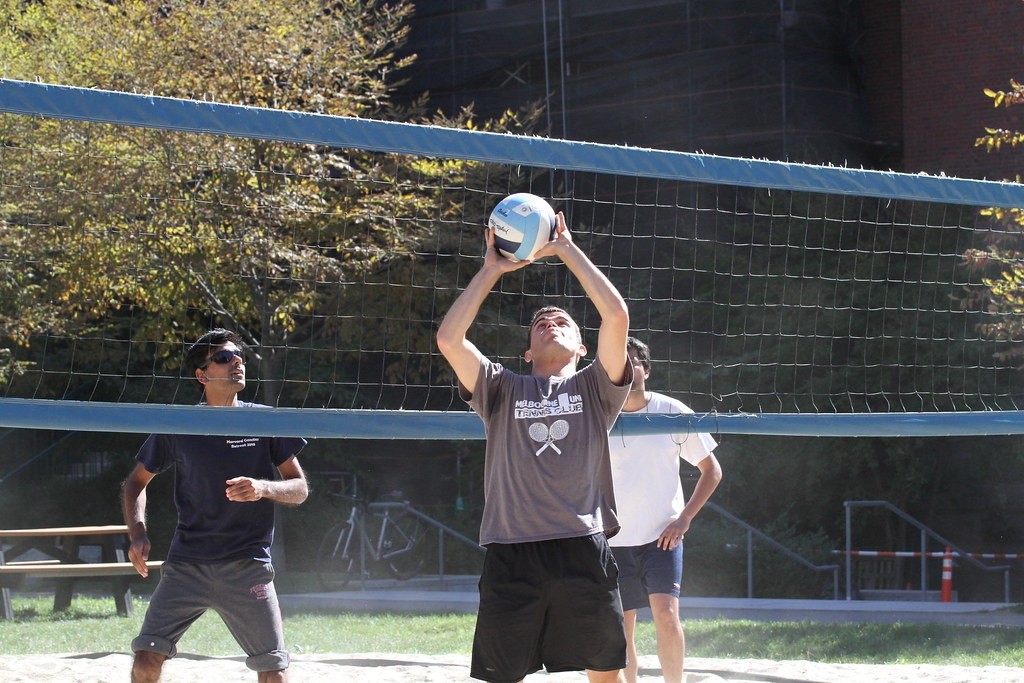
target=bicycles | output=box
[315,495,425,590]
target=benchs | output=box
[0,559,169,618]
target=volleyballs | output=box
[488,192,556,263]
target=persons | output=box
[435,210,631,683]
[598,336,724,683]
[120,328,309,683]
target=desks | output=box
[0,525,134,616]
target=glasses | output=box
[199,348,247,365]
